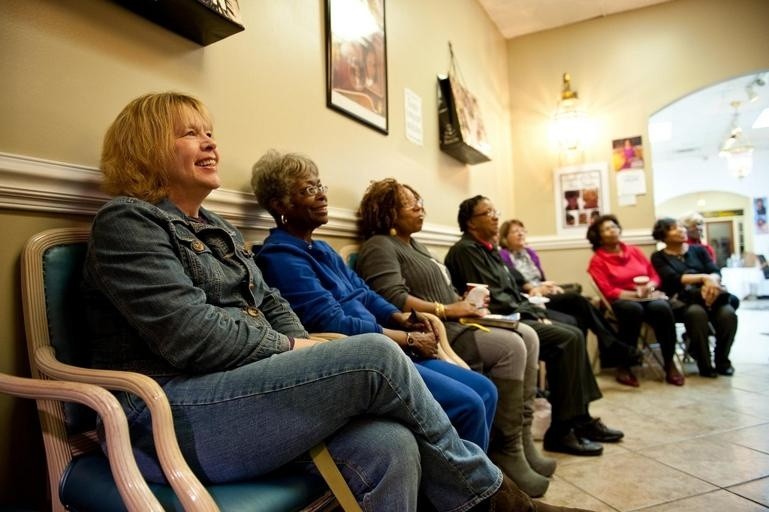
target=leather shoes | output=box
[616,358,735,388]
[543,416,625,455]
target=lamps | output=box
[560,73,579,106]
[719,100,754,181]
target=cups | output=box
[633,275,650,299]
[465,283,490,311]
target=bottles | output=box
[530,277,540,304]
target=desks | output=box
[721,266,767,301]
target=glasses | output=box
[475,208,501,216]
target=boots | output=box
[488,363,557,497]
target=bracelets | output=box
[287,336,295,351]
[435,301,447,320]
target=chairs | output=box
[674,322,717,362]
[0,373,166,511]
[587,270,667,382]
[338,242,472,369]
[245,240,460,366]
[539,361,549,392]
[19,226,344,511]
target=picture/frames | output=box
[552,161,610,239]
[324,0,389,135]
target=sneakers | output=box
[478,479,596,511]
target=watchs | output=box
[407,332,415,346]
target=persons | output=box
[498,219,645,358]
[355,176,557,497]
[587,215,685,386]
[444,195,624,456]
[650,218,738,378]
[564,188,600,225]
[757,199,766,215]
[680,215,715,262]
[362,42,377,84]
[250,149,498,455]
[710,238,727,267]
[757,219,769,234]
[79,91,591,511]
